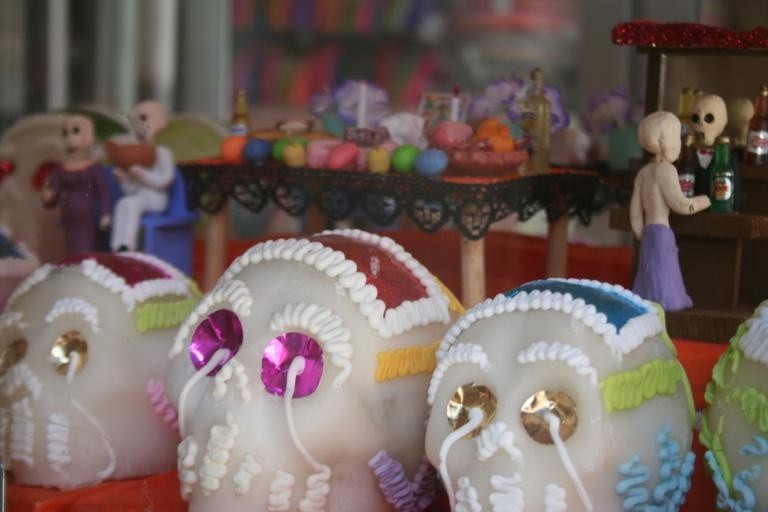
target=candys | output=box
[222,122,473,176]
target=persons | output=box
[107,95,171,248]
[40,112,112,251]
[672,90,739,304]
[625,109,714,319]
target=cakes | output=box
[0,248,204,492]
[160,227,465,512]
[423,276,698,512]
[698,301,768,510]
[1,105,139,303]
